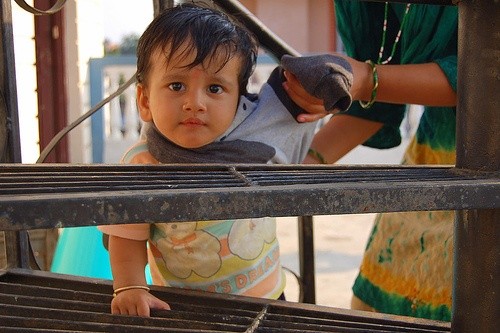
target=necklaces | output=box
[377,2,410,65]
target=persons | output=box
[298,0,458,323]
[95,3,353,317]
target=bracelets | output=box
[113,286,150,298]
[308,148,326,164]
[358,60,378,109]
